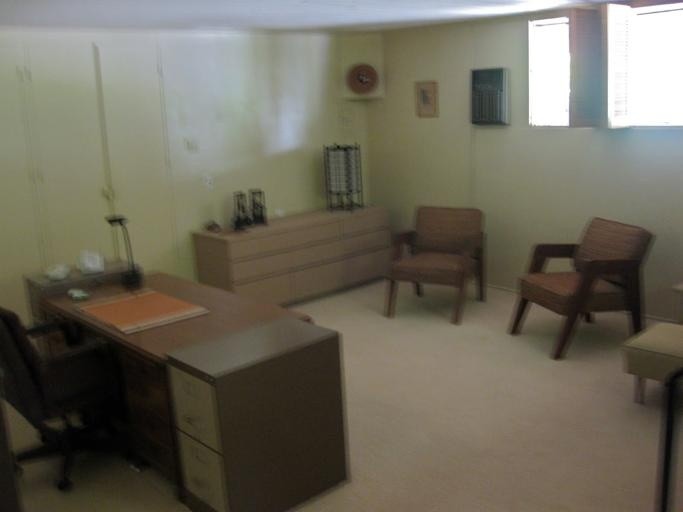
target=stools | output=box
[620,320,682,404]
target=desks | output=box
[27,271,349,512]
[191,204,394,306]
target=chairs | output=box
[506,216,653,360]
[1,308,119,493]
[380,205,486,325]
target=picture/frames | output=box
[413,80,439,119]
